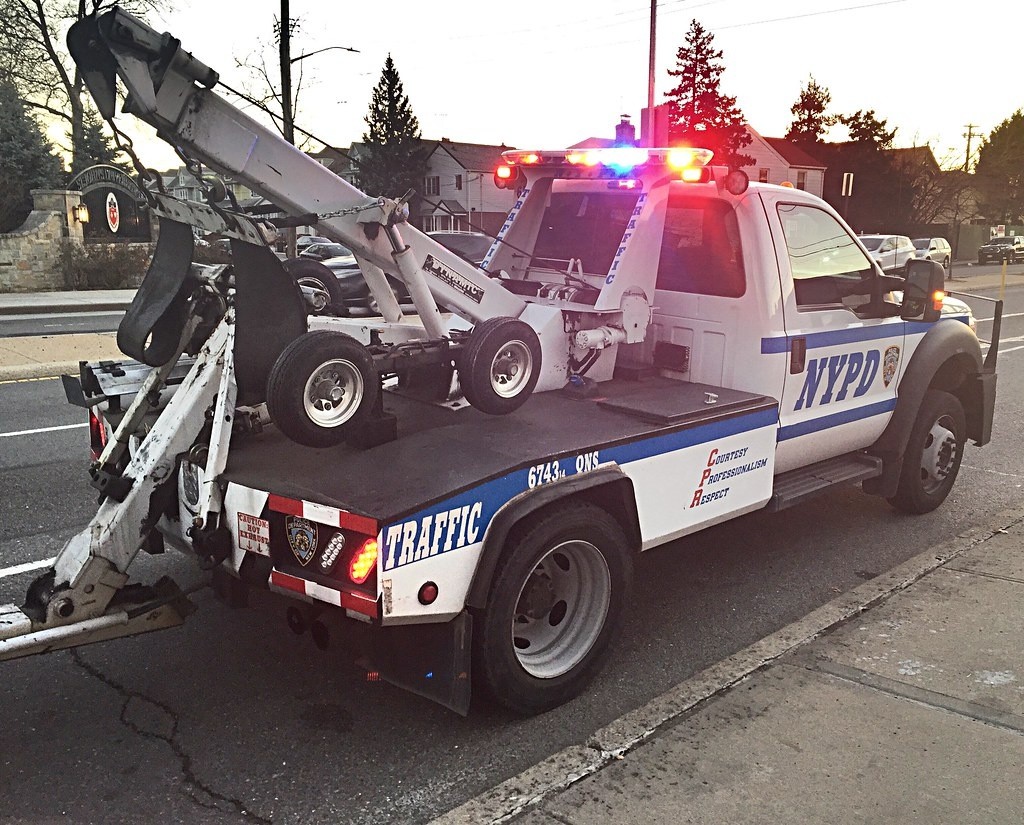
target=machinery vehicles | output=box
[0,0,1003,721]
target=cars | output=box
[299,242,352,263]
[213,239,233,257]
[285,236,331,258]
[192,232,212,250]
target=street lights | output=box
[280,46,360,262]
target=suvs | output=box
[977,236,1024,265]
[823,233,916,273]
[319,231,495,317]
[910,237,951,270]
